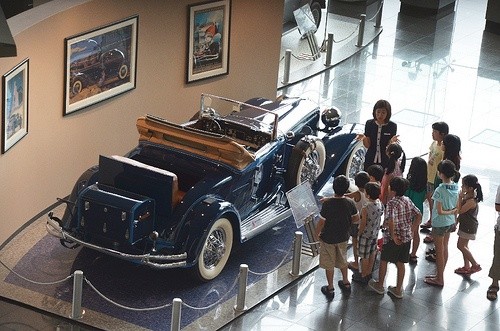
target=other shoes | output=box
[347,262,359,271]
[352,273,371,282]
[421,220,432,227]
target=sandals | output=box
[424,275,443,287]
[487,285,499,300]
[321,286,334,296]
[426,248,436,255]
[368,279,385,294]
[468,265,482,272]
[424,237,434,242]
[455,267,470,274]
[388,286,403,298]
[425,253,436,262]
[338,280,351,289]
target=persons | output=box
[366,165,384,186]
[320,171,371,270]
[380,143,406,232]
[487,185,500,300]
[368,177,422,298]
[425,134,462,262]
[420,122,449,243]
[355,100,401,171]
[352,182,385,283]
[423,160,462,287]
[455,175,483,274]
[403,157,428,264]
[315,175,360,298]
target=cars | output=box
[42,92,370,289]
[282,0,328,36]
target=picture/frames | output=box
[63,14,139,117]
[186,0,232,84]
[2,57,29,153]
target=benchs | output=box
[135,115,256,170]
